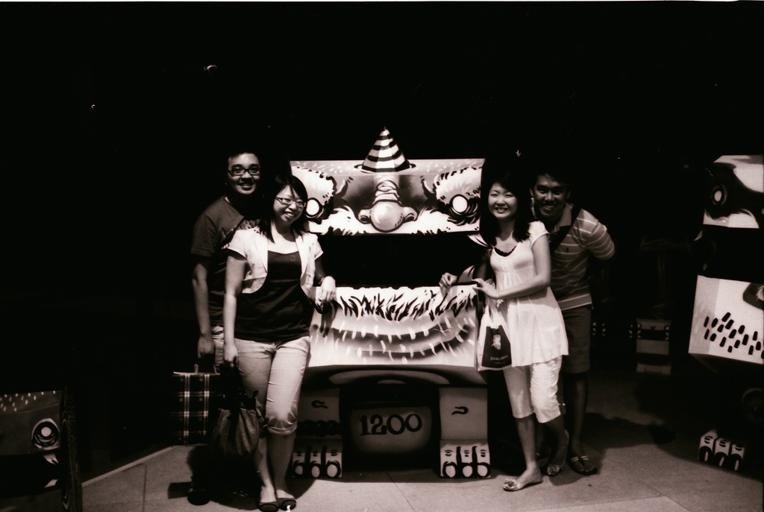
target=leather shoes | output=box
[545,431,570,475]
[503,469,540,492]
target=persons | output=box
[529,170,615,475]
[437,166,569,492]
[222,175,336,511]
[191,149,264,507]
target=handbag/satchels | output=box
[171,372,224,444]
[211,408,261,458]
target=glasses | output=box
[228,165,260,175]
[275,196,307,209]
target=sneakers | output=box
[188,478,208,502]
[280,498,297,511]
[573,455,597,475]
[259,500,279,512]
[220,483,257,509]
